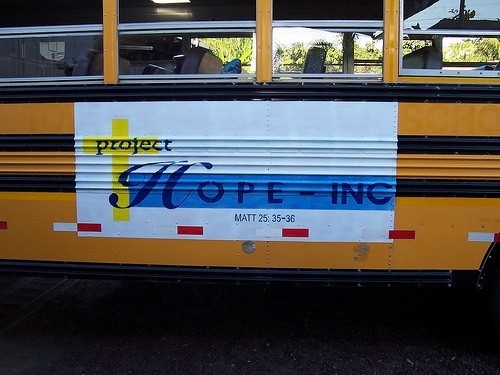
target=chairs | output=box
[181,46,222,74]
[402,46,443,69]
[299,47,326,80]
[70,48,130,75]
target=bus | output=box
[1,0,499,336]
